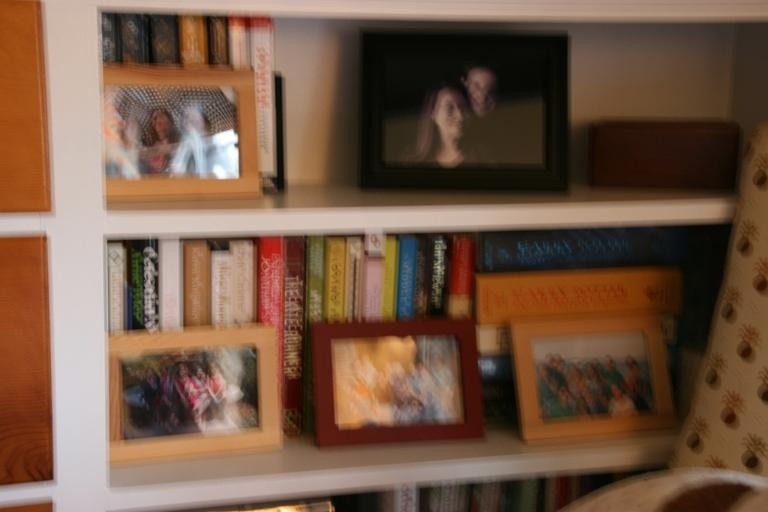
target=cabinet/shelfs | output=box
[42,1,767,511]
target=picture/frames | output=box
[509,310,679,444]
[104,324,282,466]
[358,23,573,196]
[101,62,261,200]
[309,318,486,448]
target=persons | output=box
[459,65,499,117]
[412,81,488,164]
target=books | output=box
[102,11,278,191]
